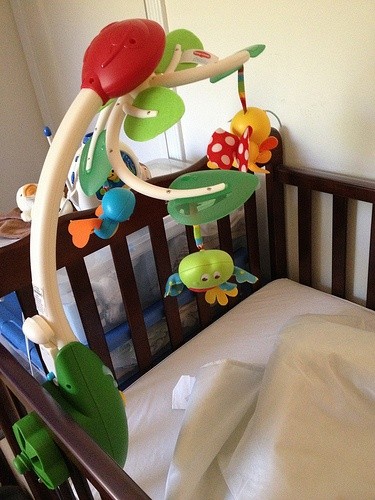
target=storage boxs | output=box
[0,249,255,398]
[0,161,250,347]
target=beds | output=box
[0,125,375,500]
